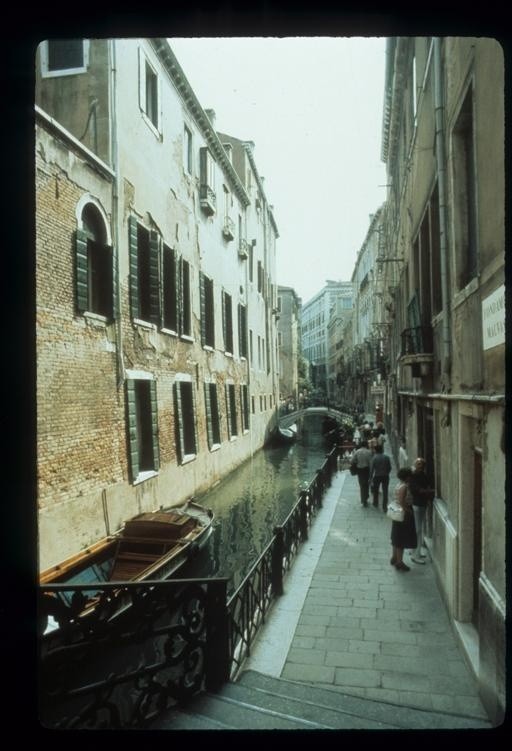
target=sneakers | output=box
[410,551,425,564]
[391,557,410,571]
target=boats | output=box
[40,496,218,644]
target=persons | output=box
[351,400,436,572]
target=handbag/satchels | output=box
[386,503,405,522]
